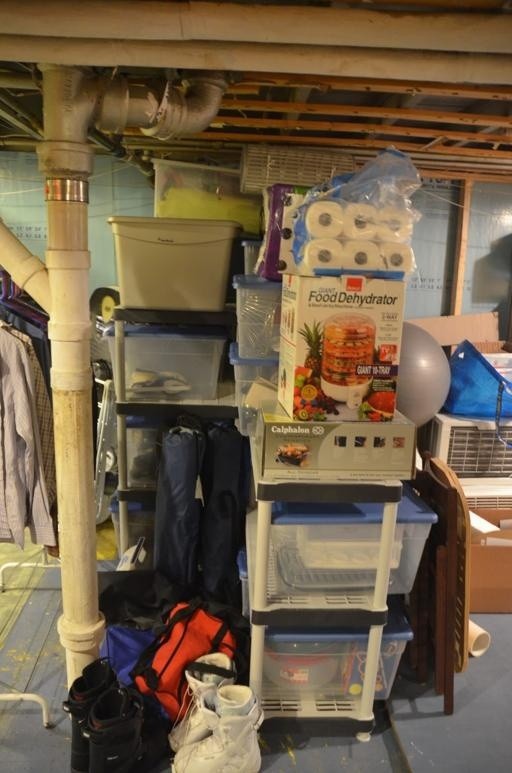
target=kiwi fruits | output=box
[298,409,309,420]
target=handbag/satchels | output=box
[98,624,168,730]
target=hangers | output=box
[0,269,49,329]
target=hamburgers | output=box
[358,391,395,421]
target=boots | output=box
[168,652,236,754]
[61,655,177,772]
[171,684,265,773]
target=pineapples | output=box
[304,355,321,377]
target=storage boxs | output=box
[470,507,512,614]
[402,312,512,358]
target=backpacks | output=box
[129,596,238,726]
